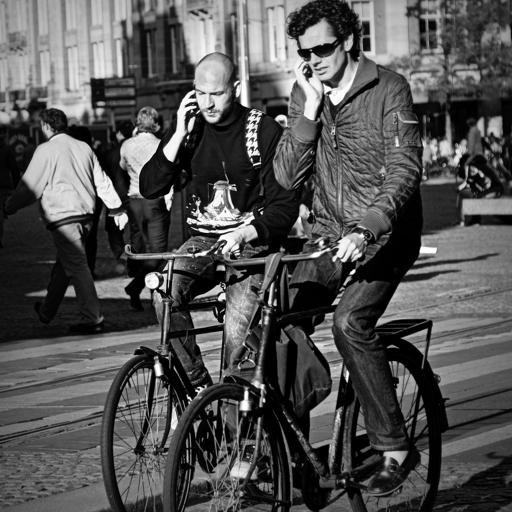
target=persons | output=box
[1,101,181,333]
[271,0,424,495]
[416,112,512,198]
[140,52,303,478]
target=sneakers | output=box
[33,299,52,324]
[125,286,144,312]
[71,324,102,334]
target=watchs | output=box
[351,224,375,245]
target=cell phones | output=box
[303,64,312,79]
[188,95,196,117]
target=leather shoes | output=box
[366,447,421,496]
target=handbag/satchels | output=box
[221,327,332,437]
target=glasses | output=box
[296,38,339,61]
